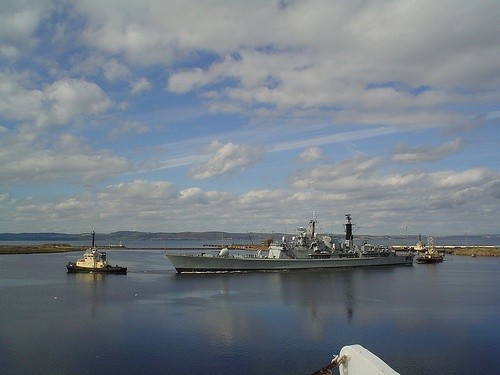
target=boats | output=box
[414,244,444,265]
[65,231,127,276]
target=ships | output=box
[166,212,415,275]
